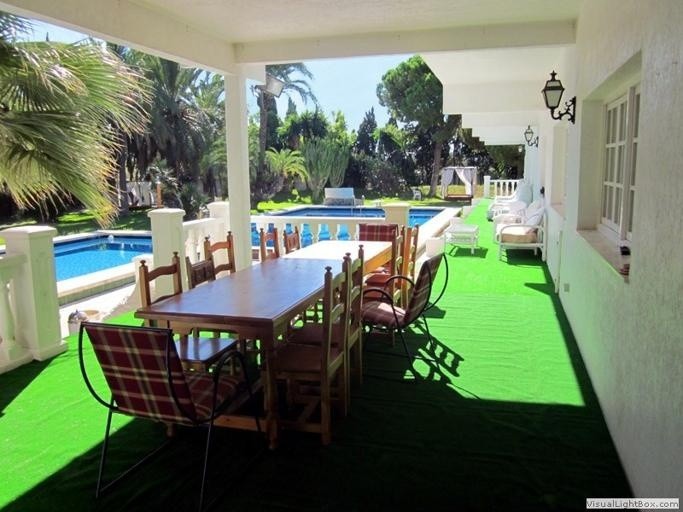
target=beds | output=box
[440,166,476,204]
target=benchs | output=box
[324,187,355,205]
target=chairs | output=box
[487,178,548,262]
[412,188,421,200]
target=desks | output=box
[445,223,479,255]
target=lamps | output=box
[541,70,577,124]
[254,72,285,97]
[524,125,539,147]
